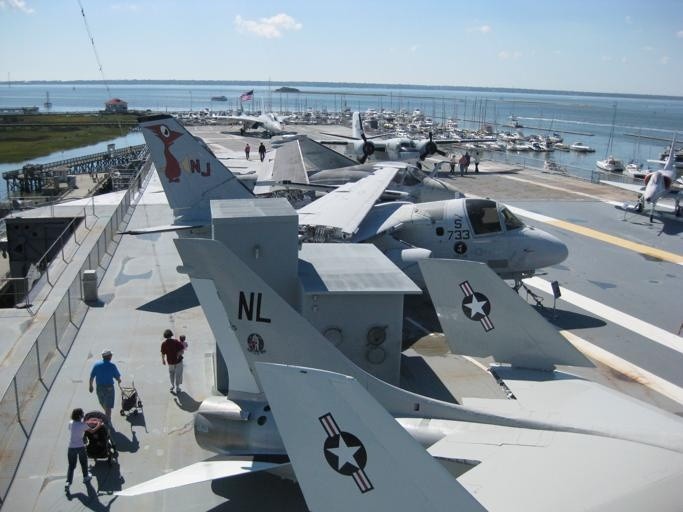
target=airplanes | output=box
[600,135,683,219]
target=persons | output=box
[258,142,266,162]
[159,329,183,394]
[244,143,250,161]
[463,152,470,167]
[86,348,120,423]
[177,334,189,351]
[63,408,100,493]
[472,153,480,174]
[449,155,458,174]
[459,154,467,177]
[126,146,134,154]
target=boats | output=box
[645,151,682,168]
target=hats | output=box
[102,349,113,357]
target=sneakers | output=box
[82,473,92,483]
[65,482,70,492]
[170,385,181,392]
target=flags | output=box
[239,90,254,103]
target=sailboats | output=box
[596,103,623,171]
[625,129,650,180]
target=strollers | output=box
[84,412,118,466]
